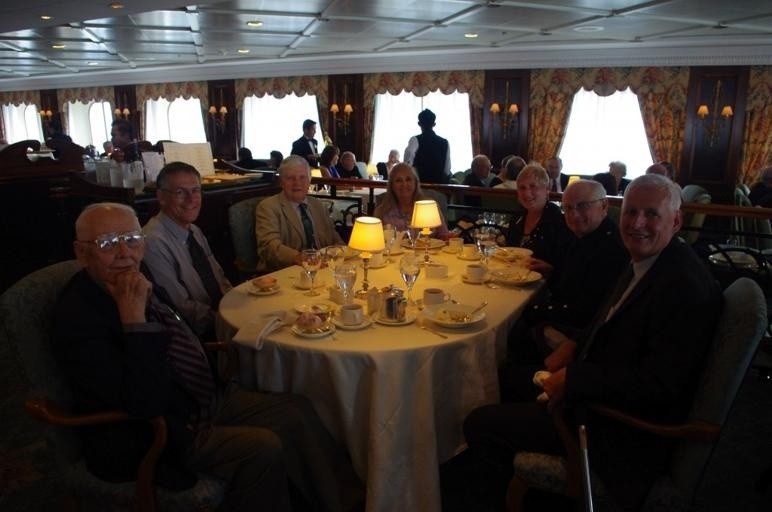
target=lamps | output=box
[114,108,130,121]
[39,110,53,121]
[209,106,228,127]
[329,104,354,128]
[697,105,734,138]
[410,200,443,267]
[347,216,386,300]
[490,103,518,128]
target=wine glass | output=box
[477,229,497,268]
[399,255,422,304]
[334,261,359,304]
[326,244,344,286]
[380,225,397,264]
[302,249,321,297]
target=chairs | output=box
[355,161,370,179]
[0,260,230,511]
[448,168,497,184]
[376,189,448,223]
[227,195,273,286]
[681,183,771,269]
[512,270,772,512]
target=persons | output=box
[372,163,457,240]
[508,180,631,363]
[506,164,574,266]
[41,117,142,163]
[463,173,725,511]
[227,109,772,211]
[51,202,364,512]
[137,161,234,339]
[252,155,347,279]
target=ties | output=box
[188,229,223,311]
[146,296,212,414]
[574,265,634,367]
[299,202,317,252]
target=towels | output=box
[231,309,288,351]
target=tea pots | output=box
[381,288,408,320]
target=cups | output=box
[462,244,476,257]
[449,237,462,253]
[297,270,311,289]
[466,264,484,281]
[421,289,453,305]
[371,175,384,181]
[342,305,364,324]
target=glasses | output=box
[561,199,603,214]
[162,188,201,199]
[75,228,147,251]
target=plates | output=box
[244,277,282,296]
[462,273,492,283]
[335,318,373,329]
[401,238,444,249]
[321,246,360,260]
[458,252,482,261]
[425,301,487,329]
[416,298,459,311]
[490,247,533,261]
[292,302,336,317]
[291,323,335,338]
[375,315,417,326]
[493,268,543,285]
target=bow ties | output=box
[306,139,315,141]
